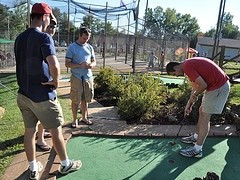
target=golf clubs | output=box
[168,102,195,145]
[82,76,94,130]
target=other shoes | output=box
[81,119,92,125]
[36,131,51,138]
[36,143,51,151]
[72,119,78,128]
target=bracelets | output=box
[193,94,197,98]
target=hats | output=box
[50,13,57,24]
[32,2,52,14]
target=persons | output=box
[166,57,230,157]
[148,51,164,71]
[14,3,82,179]
[65,28,96,128]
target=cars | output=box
[53,39,59,47]
[61,41,68,47]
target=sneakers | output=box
[180,146,202,157]
[181,134,198,143]
[59,159,82,174]
[30,161,43,180]
